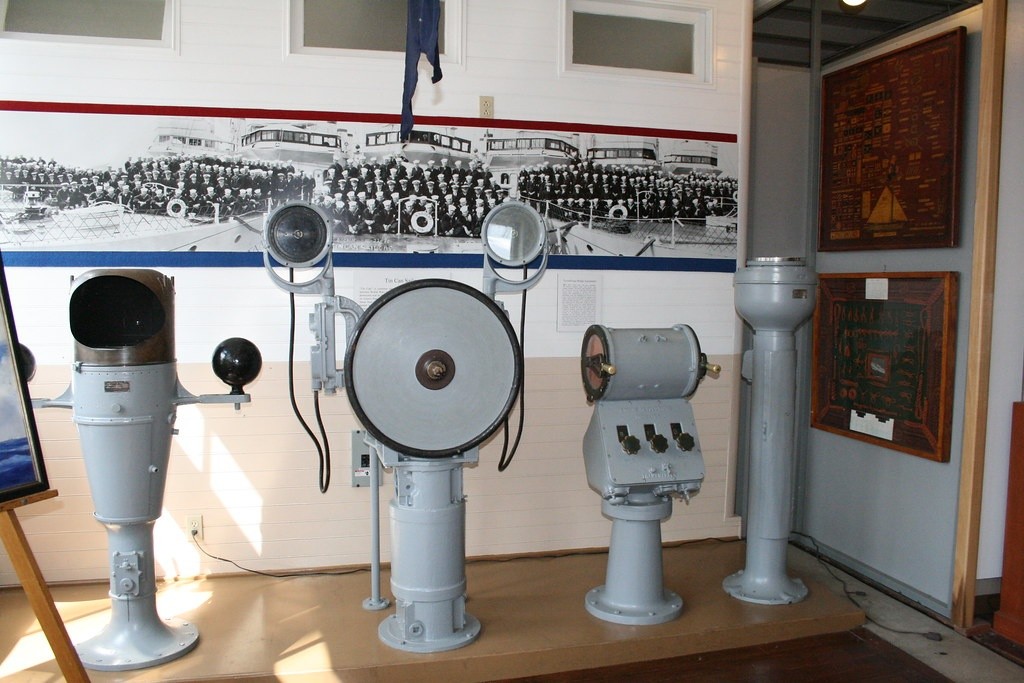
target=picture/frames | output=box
[816,24,967,254]
[809,270,960,463]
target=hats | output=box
[0,153,315,221]
[517,158,739,224]
[320,154,512,238]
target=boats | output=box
[483,132,579,167]
[52,205,124,229]
[147,125,236,158]
[238,122,343,164]
[587,135,662,169]
[664,141,722,178]
[363,126,472,164]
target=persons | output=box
[0,152,739,238]
